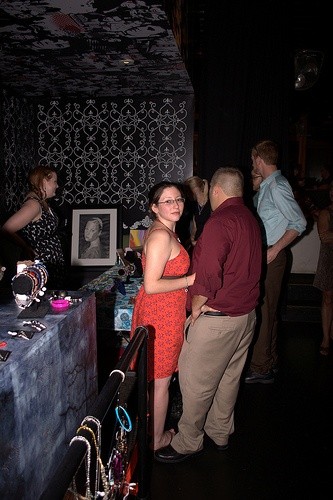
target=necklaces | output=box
[156,218,181,242]
[49,207,54,216]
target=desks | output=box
[0,291,99,500]
[43,267,145,344]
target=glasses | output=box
[153,198,185,205]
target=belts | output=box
[201,311,228,316]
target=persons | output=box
[0,166,68,290]
[154,167,262,463]
[81,218,104,258]
[313,182,333,357]
[131,181,196,454]
[175,139,307,384]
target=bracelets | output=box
[184,277,188,293]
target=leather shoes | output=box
[240,369,277,384]
[153,446,193,464]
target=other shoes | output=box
[319,346,330,357]
[154,429,175,451]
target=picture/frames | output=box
[66,204,124,272]
